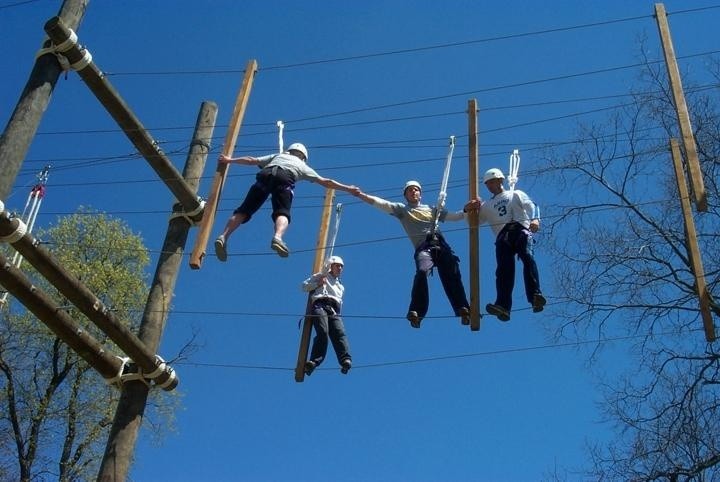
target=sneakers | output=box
[214,233,229,262]
[340,356,353,375]
[407,309,422,328]
[459,304,473,325]
[272,236,291,258]
[530,292,548,313]
[485,301,512,322]
[306,360,317,375]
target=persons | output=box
[214,142,360,262]
[350,180,479,327]
[464,167,547,321]
[302,255,352,375]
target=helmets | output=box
[483,168,507,185]
[287,141,309,160]
[326,255,346,270]
[403,179,421,193]
